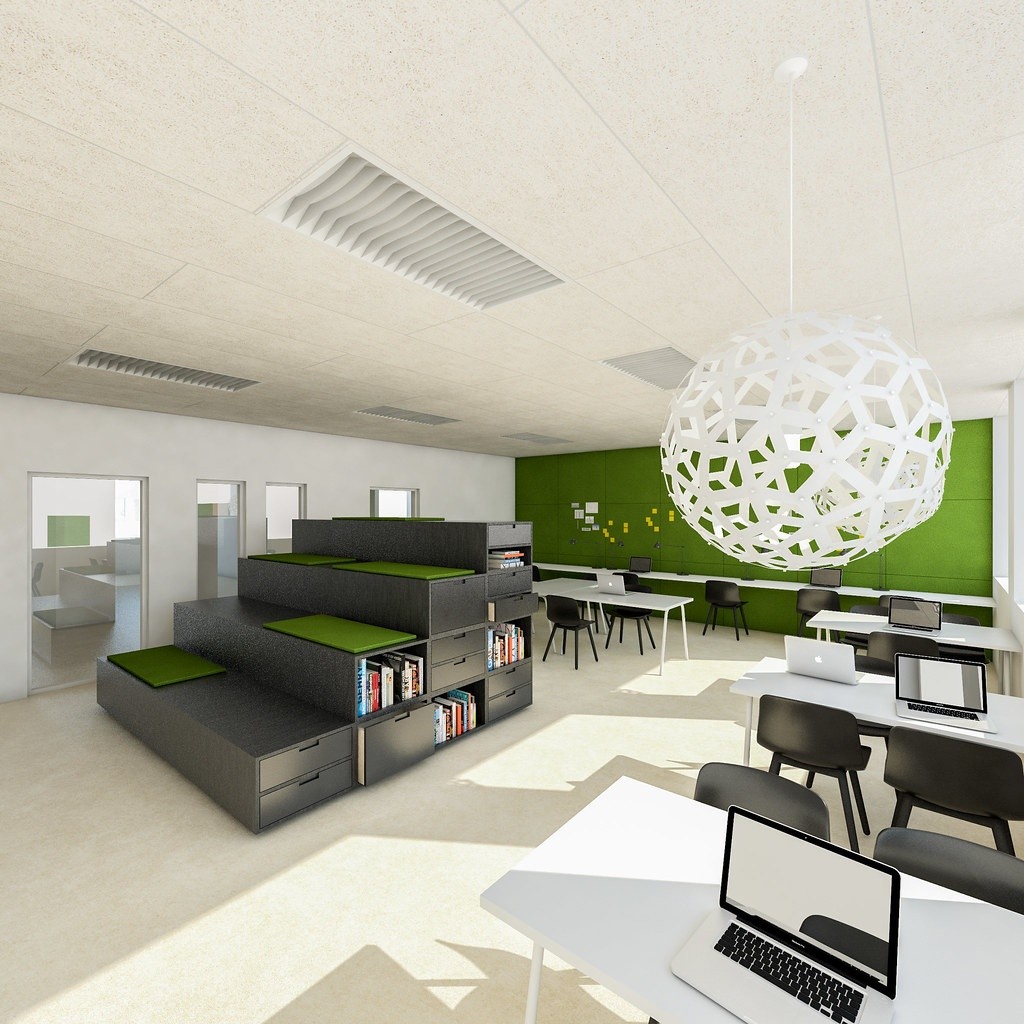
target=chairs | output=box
[842,604,888,654]
[883,726,1024,859]
[694,762,831,844]
[855,655,896,749]
[542,594,600,671]
[88,558,107,565]
[603,585,656,655]
[797,588,841,643]
[580,575,611,634]
[872,827,1024,916]
[755,694,872,857]
[32,561,44,597]
[702,579,750,640]
[879,595,924,608]
[867,631,940,663]
[937,613,991,665]
[610,572,651,622]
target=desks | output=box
[532,562,998,642]
[805,609,1024,695]
[528,577,599,652]
[559,587,694,678]
[729,656,1024,767]
[481,776,1024,1024]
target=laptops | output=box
[804,567,842,589]
[882,597,942,636]
[784,635,865,686]
[597,573,634,597]
[894,652,1001,735]
[625,556,651,575]
[668,804,903,1024]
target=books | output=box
[434,690,476,744]
[358,652,423,718]
[488,624,524,670]
[488,551,524,569]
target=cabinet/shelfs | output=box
[107,536,141,572]
[293,518,535,574]
[487,659,533,724]
[57,562,142,619]
[485,567,540,622]
[31,594,115,670]
[430,676,488,751]
[96,647,357,838]
[430,626,487,693]
[174,596,430,721]
[356,697,436,788]
[236,554,487,639]
[485,615,532,674]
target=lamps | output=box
[657,57,956,573]
